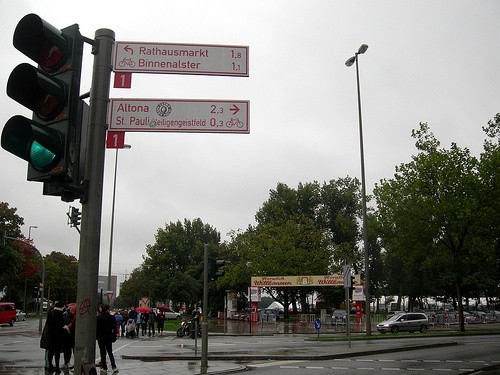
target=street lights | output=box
[345,43,373,335]
[23,225,38,313]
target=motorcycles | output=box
[177,319,202,338]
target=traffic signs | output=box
[107,98,250,134]
[112,40,250,77]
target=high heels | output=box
[56,368,61,374]
[48,368,54,374]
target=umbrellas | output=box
[137,306,154,318]
[158,305,171,316]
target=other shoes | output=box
[67,363,73,368]
[61,365,68,369]
[70,368,75,372]
[95,362,103,366]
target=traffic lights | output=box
[70,207,79,223]
[74,212,81,226]
[0,229,7,249]
[1,13,84,182]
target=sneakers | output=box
[113,368,120,372]
[100,368,107,373]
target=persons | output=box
[188,308,200,334]
[68,307,76,372]
[49,302,72,373]
[41,304,56,370]
[141,311,149,336]
[149,308,158,335]
[97,304,121,372]
[444,309,449,325]
[114,307,142,338]
[97,305,107,366]
[432,311,437,325]
[157,311,165,336]
[59,305,76,371]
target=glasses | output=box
[108,309,111,310]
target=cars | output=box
[376,312,429,334]
[331,310,347,324]
[155,307,182,320]
[386,311,406,319]
[16,309,26,321]
[0,302,16,326]
